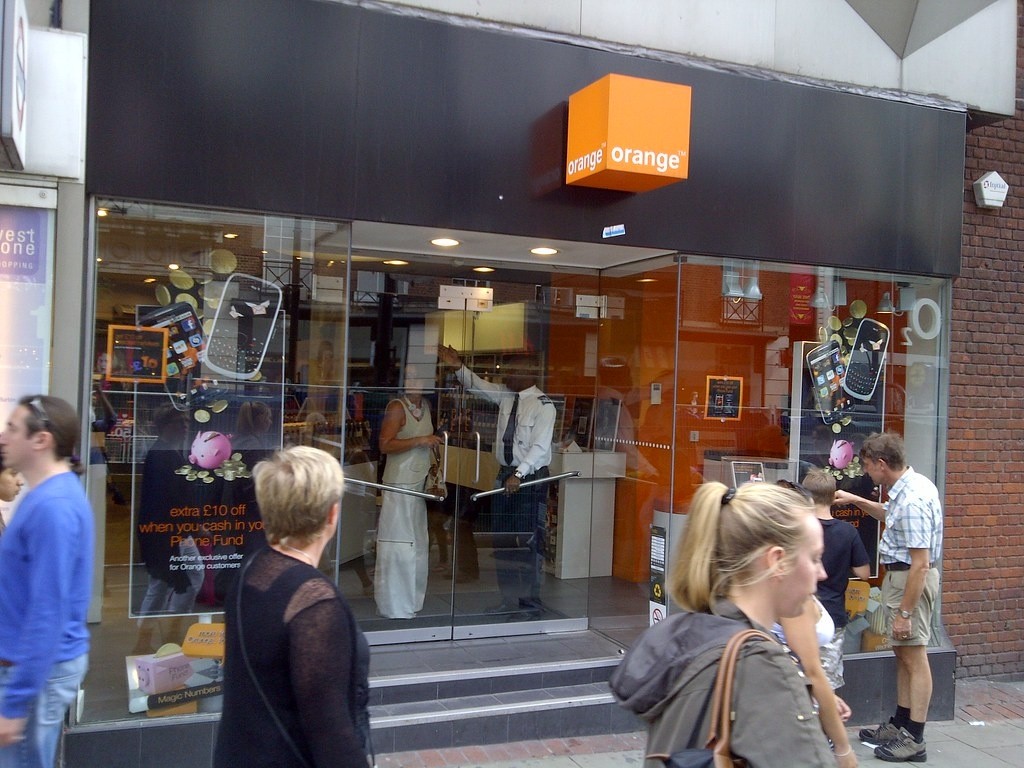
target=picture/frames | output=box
[541,392,622,453]
[704,374,743,421]
[108,324,169,383]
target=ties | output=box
[502,393,521,465]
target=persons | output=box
[90,389,119,464]
[435,341,557,620]
[211,445,371,768]
[609,479,837,768]
[770,481,860,768]
[373,356,444,618]
[833,431,943,762]
[801,471,871,640]
[597,358,698,483]
[94,347,123,391]
[220,400,272,554]
[0,394,96,768]
[131,402,212,655]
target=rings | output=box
[903,636,907,638]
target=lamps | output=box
[721,267,894,314]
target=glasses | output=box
[23,394,53,433]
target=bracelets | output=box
[834,745,851,757]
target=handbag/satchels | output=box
[640,629,784,768]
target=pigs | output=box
[829,439,854,469]
[188,430,233,468]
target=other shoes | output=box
[506,606,543,623]
[483,602,515,618]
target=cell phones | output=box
[804,339,857,425]
[842,318,890,401]
[205,273,283,379]
[137,301,230,411]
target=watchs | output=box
[898,608,911,619]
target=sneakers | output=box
[858,719,902,747]
[872,726,928,764]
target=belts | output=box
[885,561,938,572]
[0,659,14,670]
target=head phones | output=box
[404,395,424,417]
